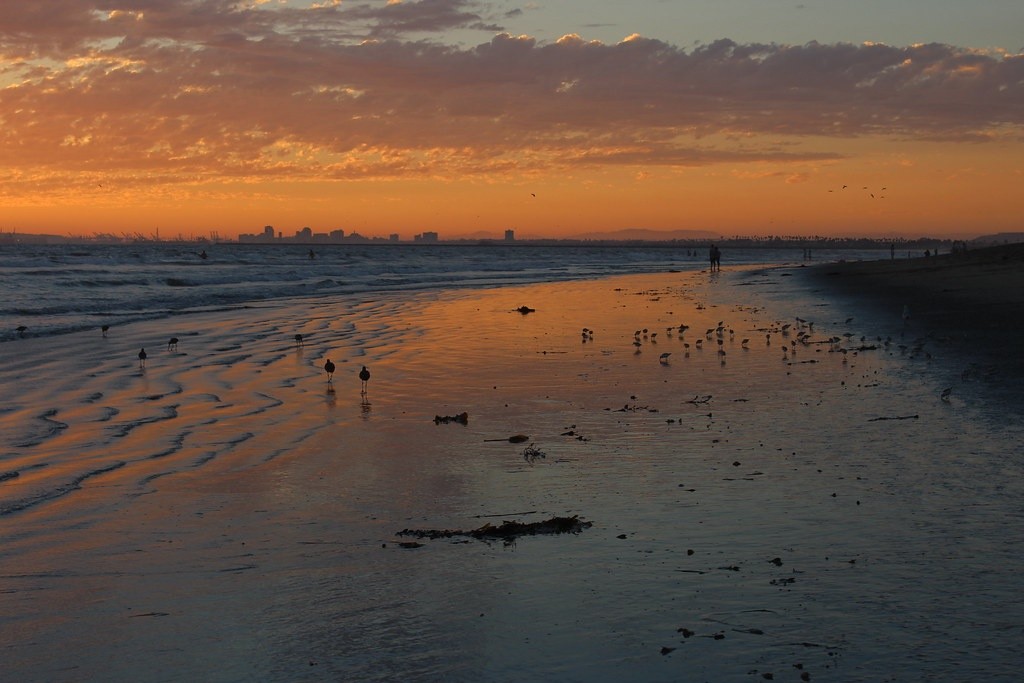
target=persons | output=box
[200,251,208,259]
[308,249,316,260]
[889,240,968,261]
[803,248,812,259]
[687,244,722,273]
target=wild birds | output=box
[15,326,28,333]
[359,366,371,392]
[101,324,110,336]
[827,184,886,199]
[168,338,179,348]
[295,334,303,346]
[325,358,335,383]
[138,348,147,364]
[581,314,972,401]
